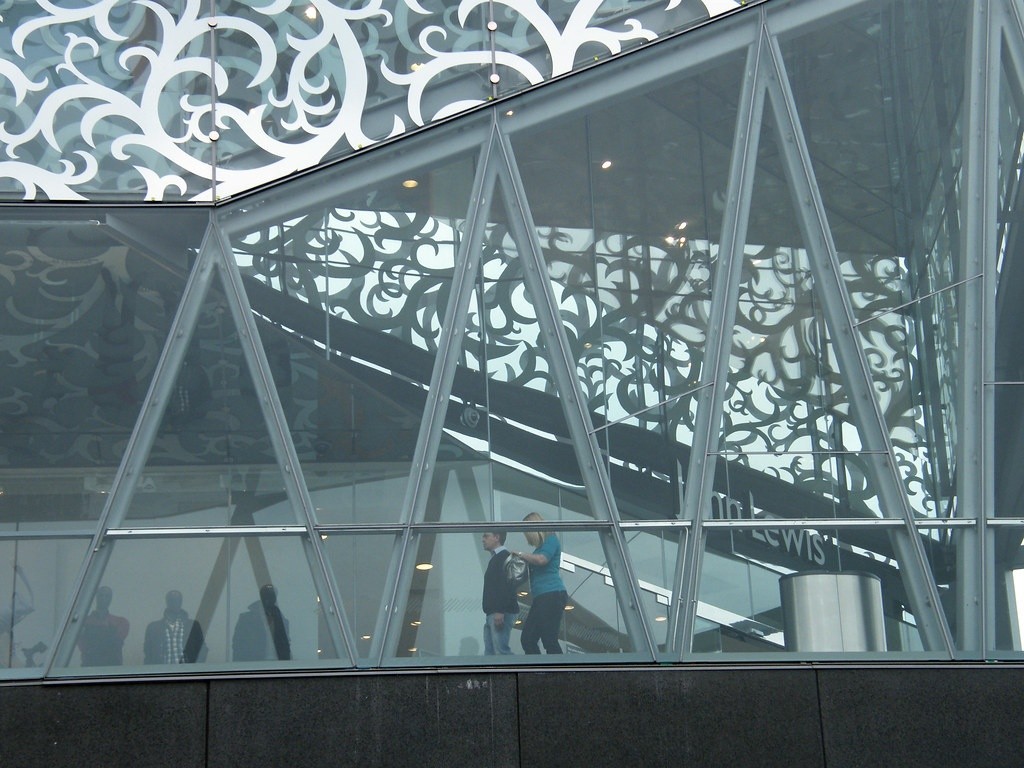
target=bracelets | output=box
[517,550,524,560]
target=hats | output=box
[261,585,276,594]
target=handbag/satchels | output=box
[504,553,531,592]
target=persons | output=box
[483,531,520,656]
[77,586,129,668]
[143,590,210,664]
[231,585,294,661]
[510,513,568,654]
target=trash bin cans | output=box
[778,570,890,654]
